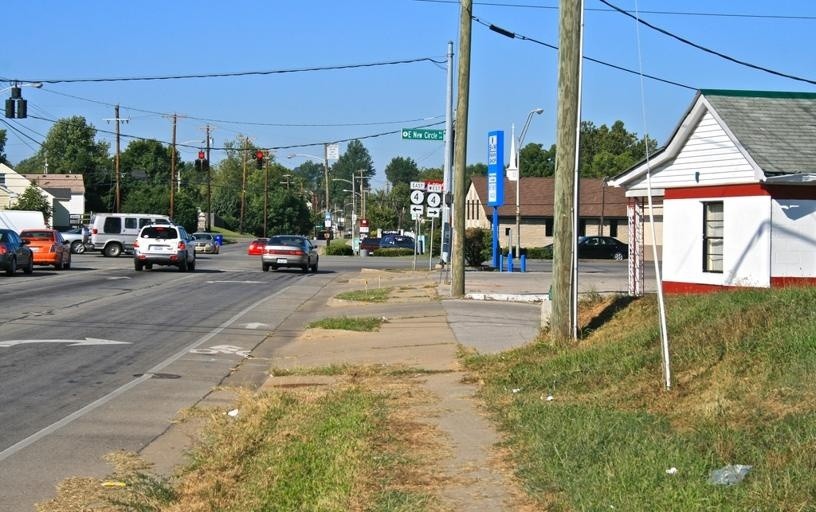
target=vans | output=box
[361,235,415,250]
[88,211,180,257]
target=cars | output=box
[578,236,629,262]
[191,233,219,253]
[58,224,90,254]
[261,235,318,274]
[247,238,270,255]
[0,228,33,276]
[19,229,72,270]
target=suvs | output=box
[132,222,198,273]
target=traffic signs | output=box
[400,128,444,141]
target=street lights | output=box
[280,153,376,250]
[1,83,43,94]
[176,140,205,193]
[516,108,545,260]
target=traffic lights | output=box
[194,150,210,172]
[256,151,263,170]
